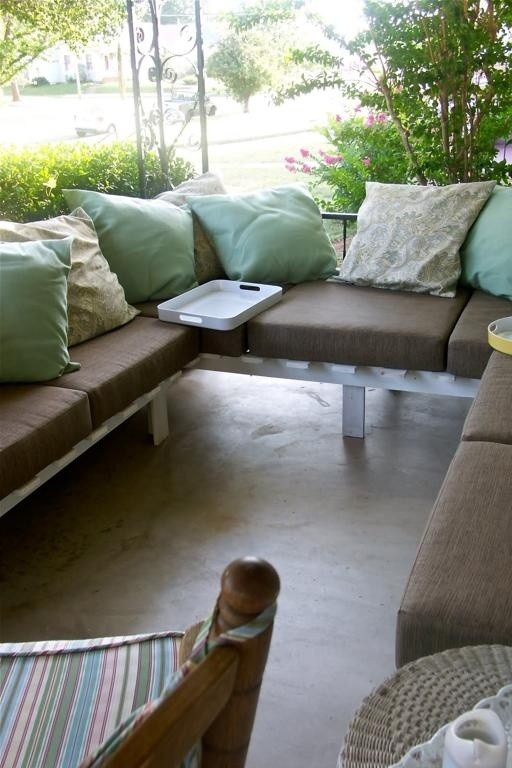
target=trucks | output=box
[156,82,219,122]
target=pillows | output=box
[461,185,510,299]
[0,234,81,384]
[61,186,199,303]
[151,171,227,285]
[193,182,340,289]
[332,180,497,299]
[0,204,142,346]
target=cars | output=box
[66,88,143,137]
